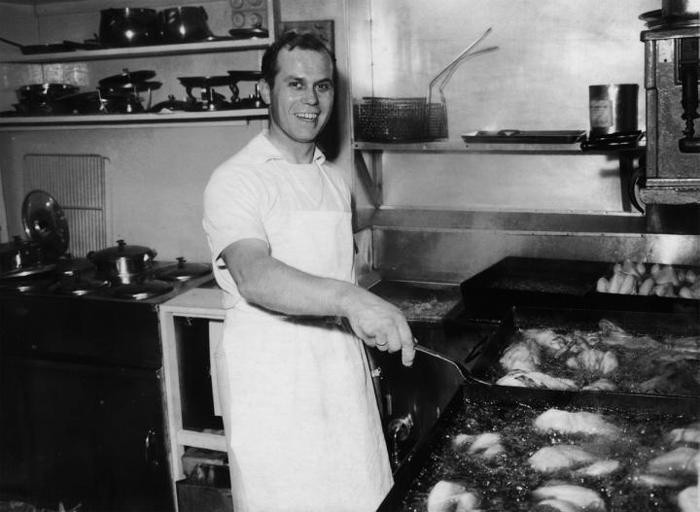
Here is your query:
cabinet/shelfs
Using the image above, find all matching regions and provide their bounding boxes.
[0,0,700,512]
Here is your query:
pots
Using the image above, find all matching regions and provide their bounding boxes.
[0,36,75,54]
[87,239,157,272]
[1,234,39,275]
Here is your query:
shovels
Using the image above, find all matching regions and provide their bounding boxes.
[414,343,495,386]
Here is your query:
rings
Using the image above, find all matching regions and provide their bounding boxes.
[375,339,390,348]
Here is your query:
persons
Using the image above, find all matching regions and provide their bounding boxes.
[200,30,421,511]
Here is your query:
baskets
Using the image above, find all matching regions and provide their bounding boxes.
[423,101,449,141]
[352,95,428,143]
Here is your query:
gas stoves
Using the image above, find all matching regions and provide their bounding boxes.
[32,255,214,299]
